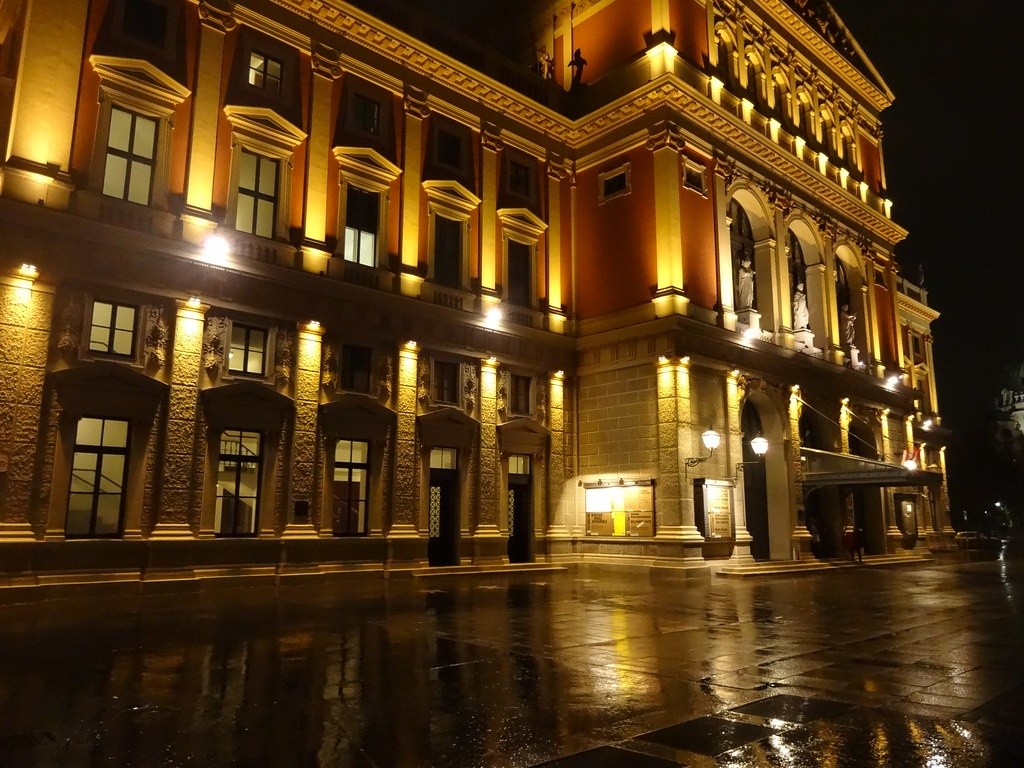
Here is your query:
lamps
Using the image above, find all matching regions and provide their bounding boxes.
[737,430,770,471]
[685,426,721,467]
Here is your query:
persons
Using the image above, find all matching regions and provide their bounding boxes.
[793,282,809,330]
[739,257,754,308]
[849,525,864,562]
[840,305,857,346]
